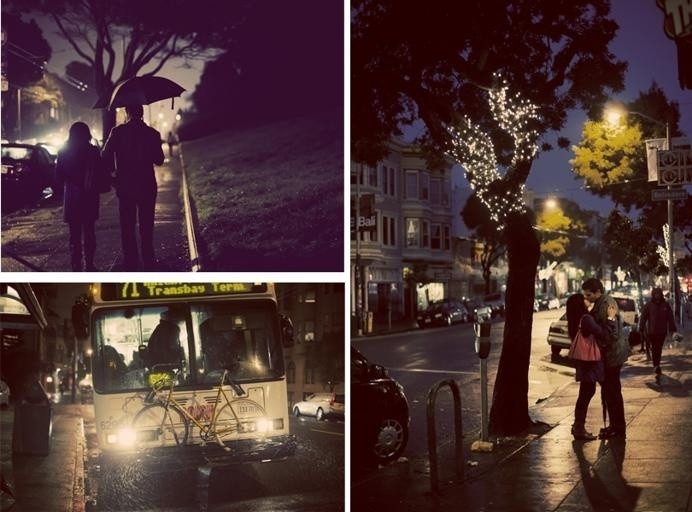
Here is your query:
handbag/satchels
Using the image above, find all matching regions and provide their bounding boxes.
[568,313,602,362]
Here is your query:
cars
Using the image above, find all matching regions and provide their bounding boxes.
[291,392,344,422]
[416,291,506,330]
[548,290,648,360]
[1,141,58,205]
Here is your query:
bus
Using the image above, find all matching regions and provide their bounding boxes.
[69,282,291,475]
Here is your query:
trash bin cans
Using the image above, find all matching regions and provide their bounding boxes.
[14,380,51,457]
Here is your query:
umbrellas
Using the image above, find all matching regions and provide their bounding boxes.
[89,74,185,113]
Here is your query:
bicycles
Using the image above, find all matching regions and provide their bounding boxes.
[129,359,273,457]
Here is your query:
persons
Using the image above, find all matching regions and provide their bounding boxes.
[50,119,114,271]
[97,102,166,274]
[639,287,680,376]
[580,276,634,441]
[166,130,177,158]
[144,303,187,380]
[564,293,620,445]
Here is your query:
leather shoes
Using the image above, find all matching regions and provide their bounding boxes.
[598,427,626,439]
[570,424,596,442]
